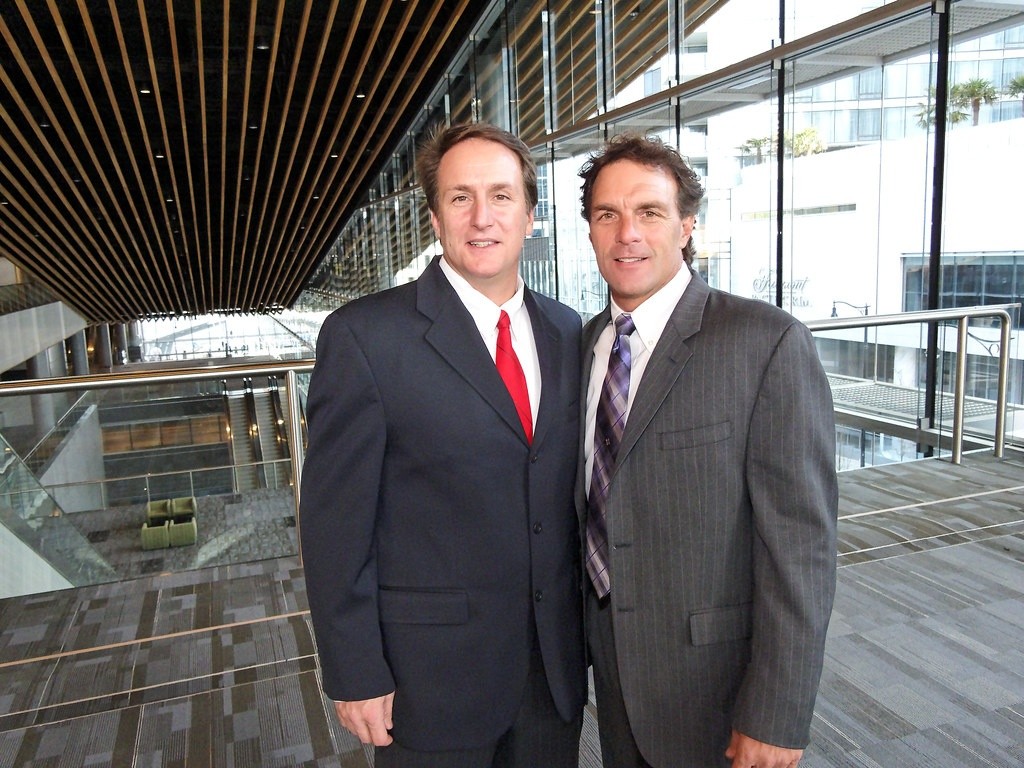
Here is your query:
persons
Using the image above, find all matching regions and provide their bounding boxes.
[300,122,593,768]
[576,131,839,768]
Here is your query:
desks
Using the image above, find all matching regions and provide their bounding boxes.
[151,515,190,527]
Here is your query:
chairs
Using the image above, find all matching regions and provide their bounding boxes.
[140,521,169,550]
[169,516,198,548]
[172,497,198,518]
[147,499,171,525]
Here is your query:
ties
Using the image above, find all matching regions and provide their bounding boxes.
[495,310,533,448]
[585,313,636,600]
